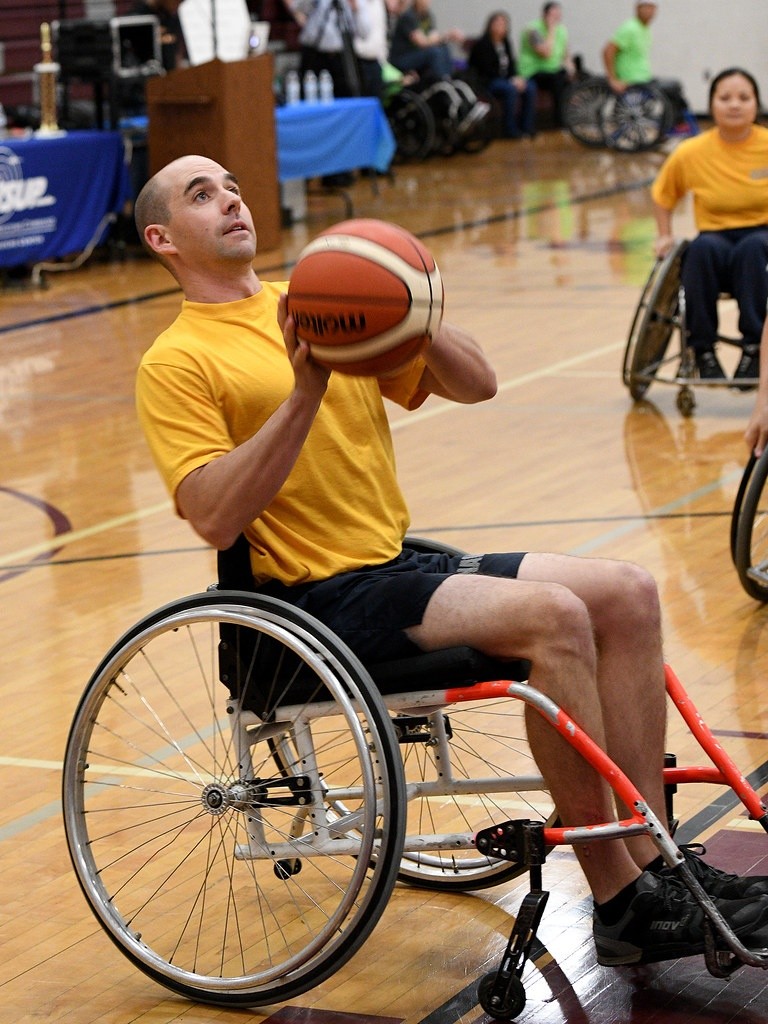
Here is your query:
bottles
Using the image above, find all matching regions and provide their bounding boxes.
[284,71,300,104]
[304,70,318,102]
[319,70,335,106]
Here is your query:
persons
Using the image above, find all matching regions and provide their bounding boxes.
[464,9,537,140]
[602,0,690,132]
[743,314,768,456]
[289,0,467,189]
[517,2,573,131]
[651,67,768,392]
[131,154,768,965]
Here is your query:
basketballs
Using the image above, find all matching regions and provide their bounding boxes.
[284,216,446,378]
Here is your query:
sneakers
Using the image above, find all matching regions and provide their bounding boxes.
[593,871,768,967]
[655,843,768,900]
[730,348,759,392]
[696,349,725,377]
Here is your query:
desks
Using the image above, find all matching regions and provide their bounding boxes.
[118,98,380,224]
[0,128,121,284]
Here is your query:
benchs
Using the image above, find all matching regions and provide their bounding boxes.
[465,38,553,132]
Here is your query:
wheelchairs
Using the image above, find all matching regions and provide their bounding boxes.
[62,533,768,1020]
[380,78,494,158]
[569,75,697,154]
[622,239,768,415]
[727,433,768,611]
